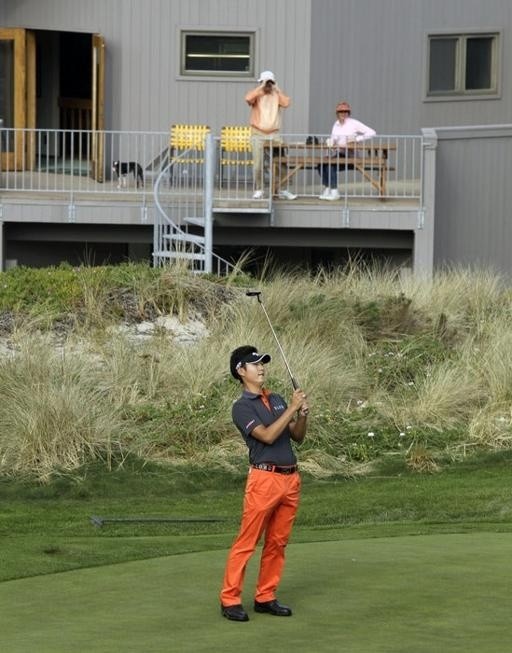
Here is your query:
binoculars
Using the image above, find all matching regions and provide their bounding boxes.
[306,136,318,145]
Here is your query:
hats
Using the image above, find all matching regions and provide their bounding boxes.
[335,102,351,116]
[230,345,272,379]
[257,70,276,82]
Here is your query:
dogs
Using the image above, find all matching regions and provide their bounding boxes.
[112,160,144,189]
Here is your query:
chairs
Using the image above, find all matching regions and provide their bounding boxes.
[168,124,212,189]
[218,126,252,190]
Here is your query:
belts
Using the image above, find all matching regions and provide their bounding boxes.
[252,462,299,474]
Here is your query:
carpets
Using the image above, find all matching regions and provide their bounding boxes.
[41,167,91,177]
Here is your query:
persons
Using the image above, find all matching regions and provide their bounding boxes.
[246,71,376,201]
[218,345,308,622]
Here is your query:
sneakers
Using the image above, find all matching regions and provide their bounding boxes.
[252,190,266,199]
[273,190,299,200]
[318,187,340,201]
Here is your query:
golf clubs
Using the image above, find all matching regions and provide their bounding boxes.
[246,292,309,415]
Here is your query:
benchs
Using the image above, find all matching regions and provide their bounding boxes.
[264,141,399,200]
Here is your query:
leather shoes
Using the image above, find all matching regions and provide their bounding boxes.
[221,603,249,621]
[253,600,292,616]
